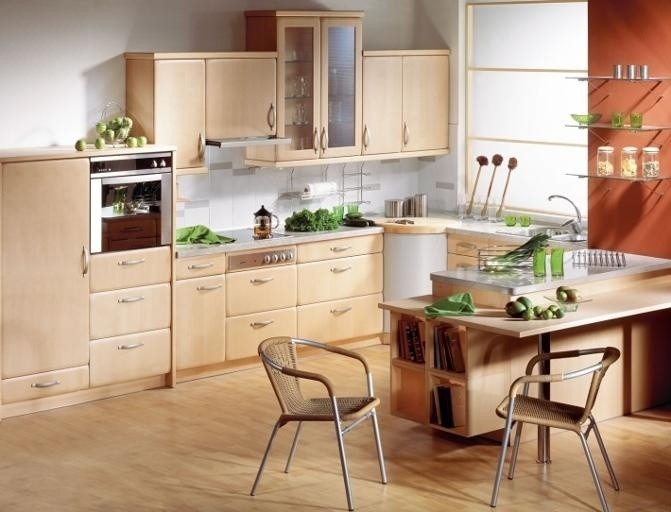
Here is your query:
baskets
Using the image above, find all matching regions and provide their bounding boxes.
[94,101,132,148]
[478,244,536,274]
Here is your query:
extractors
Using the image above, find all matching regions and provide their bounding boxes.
[207,136,294,147]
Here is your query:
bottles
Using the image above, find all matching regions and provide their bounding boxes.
[384,192,429,219]
[642,148,661,178]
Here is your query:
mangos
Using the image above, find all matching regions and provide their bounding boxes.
[516,297,532,312]
[564,289,578,302]
[556,286,571,300]
[504,301,527,317]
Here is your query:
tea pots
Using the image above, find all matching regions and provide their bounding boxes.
[254,205,280,235]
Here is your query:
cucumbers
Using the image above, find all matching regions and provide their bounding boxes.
[345,212,361,218]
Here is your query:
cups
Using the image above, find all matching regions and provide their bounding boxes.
[520,216,530,226]
[611,110,627,128]
[534,246,546,279]
[334,206,345,223]
[505,215,517,227]
[348,204,359,213]
[551,248,566,277]
[627,63,638,80]
[613,64,624,79]
[640,63,650,81]
[630,111,645,128]
[290,45,343,127]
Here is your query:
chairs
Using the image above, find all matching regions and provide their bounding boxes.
[490,346,621,512]
[247,336,390,512]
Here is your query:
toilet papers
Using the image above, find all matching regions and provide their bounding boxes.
[301,182,338,198]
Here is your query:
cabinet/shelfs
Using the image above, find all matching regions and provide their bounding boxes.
[124,52,280,177]
[390,310,513,441]
[1,156,91,418]
[91,245,173,401]
[244,10,365,168]
[447,233,527,271]
[362,49,450,161]
[564,76,670,197]
[228,267,297,372]
[176,255,233,375]
[297,232,384,354]
[101,216,161,252]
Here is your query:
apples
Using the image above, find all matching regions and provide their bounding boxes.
[75,140,86,150]
[96,117,133,140]
[127,137,137,147]
[137,136,147,147]
[95,138,105,149]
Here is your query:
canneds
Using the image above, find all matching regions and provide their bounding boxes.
[642,146,659,178]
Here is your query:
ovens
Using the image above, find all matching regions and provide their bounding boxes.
[91,150,173,254]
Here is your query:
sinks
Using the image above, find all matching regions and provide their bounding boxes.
[496,226,587,241]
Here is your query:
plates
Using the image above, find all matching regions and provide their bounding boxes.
[573,112,604,126]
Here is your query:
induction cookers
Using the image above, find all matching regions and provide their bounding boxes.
[215,227,297,273]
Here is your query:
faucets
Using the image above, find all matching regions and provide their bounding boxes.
[548,195,582,234]
